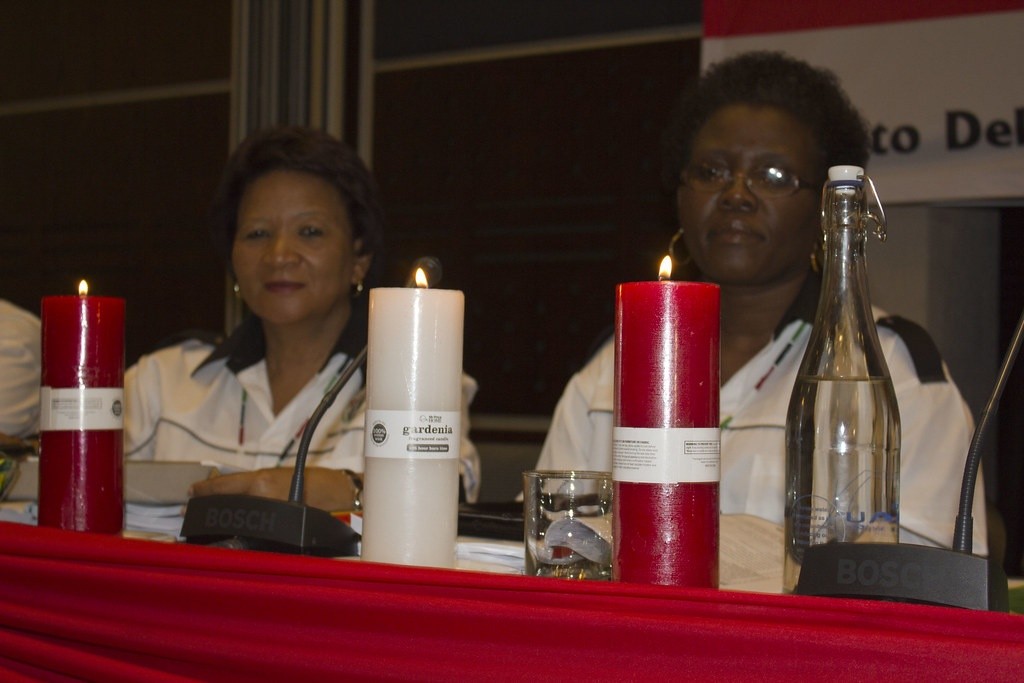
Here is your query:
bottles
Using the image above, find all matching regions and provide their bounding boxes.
[784,165,902,595]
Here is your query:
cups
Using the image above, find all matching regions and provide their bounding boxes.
[521,469,612,582]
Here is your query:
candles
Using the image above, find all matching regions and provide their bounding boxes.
[613,256,722,592]
[359,267,464,570]
[37,281,126,538]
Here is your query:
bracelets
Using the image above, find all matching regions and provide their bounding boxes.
[343,469,361,510]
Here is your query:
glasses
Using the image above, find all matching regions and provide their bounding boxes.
[671,159,822,198]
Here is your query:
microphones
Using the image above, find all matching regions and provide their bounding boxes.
[180,256,443,554]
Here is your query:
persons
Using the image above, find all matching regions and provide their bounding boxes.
[0,297,41,453]
[123,130,479,518]
[522,52,990,593]
